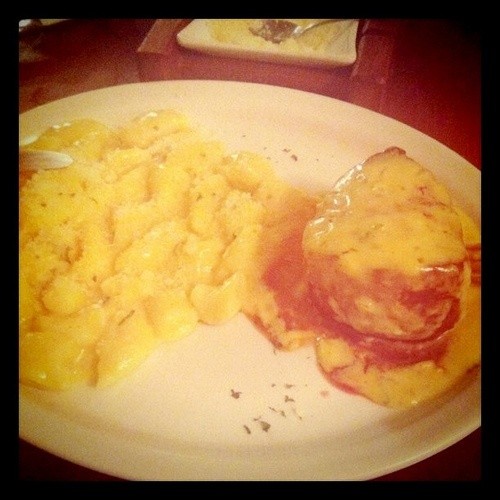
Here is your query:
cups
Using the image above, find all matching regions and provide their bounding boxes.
[19,19,43,62]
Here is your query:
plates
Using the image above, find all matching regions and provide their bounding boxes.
[18,79,482,481]
[177,19,359,66]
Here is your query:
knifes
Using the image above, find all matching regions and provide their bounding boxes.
[18,147,73,169]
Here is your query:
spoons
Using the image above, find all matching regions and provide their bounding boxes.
[248,19,350,44]
